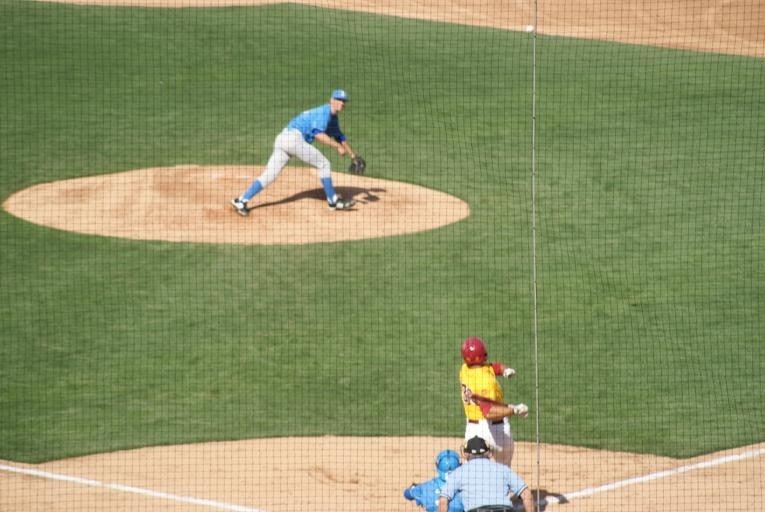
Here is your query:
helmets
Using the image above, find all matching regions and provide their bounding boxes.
[461,338,488,365]
[435,450,462,481]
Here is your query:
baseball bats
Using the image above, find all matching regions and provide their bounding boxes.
[466,393,517,410]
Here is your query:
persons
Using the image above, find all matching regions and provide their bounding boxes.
[404,449,465,512]
[438,435,535,512]
[459,338,528,469]
[230,88,365,217]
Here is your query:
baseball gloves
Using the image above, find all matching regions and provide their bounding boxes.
[347,155,367,176]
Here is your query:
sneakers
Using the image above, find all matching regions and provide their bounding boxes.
[328,198,355,210]
[230,198,248,216]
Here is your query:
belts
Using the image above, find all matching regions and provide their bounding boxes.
[466,420,505,425]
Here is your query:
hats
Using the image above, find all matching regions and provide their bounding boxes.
[332,89,348,101]
[465,435,489,455]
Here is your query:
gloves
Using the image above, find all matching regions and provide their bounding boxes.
[514,402,528,419]
[504,367,516,382]
